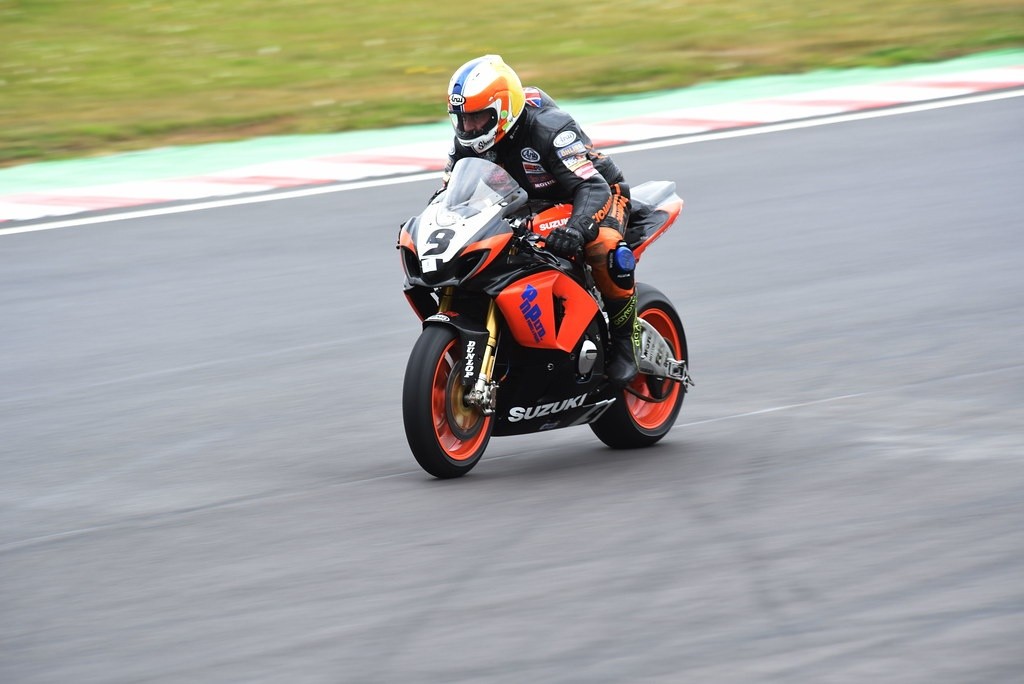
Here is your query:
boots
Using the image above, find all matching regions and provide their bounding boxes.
[601,286,641,387]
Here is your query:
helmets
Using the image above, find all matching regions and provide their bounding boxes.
[447,54,526,154]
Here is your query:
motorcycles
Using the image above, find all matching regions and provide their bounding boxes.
[396,157,693,481]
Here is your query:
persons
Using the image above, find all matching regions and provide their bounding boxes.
[426,55,642,382]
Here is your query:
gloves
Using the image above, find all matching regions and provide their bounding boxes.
[545,215,599,262]
